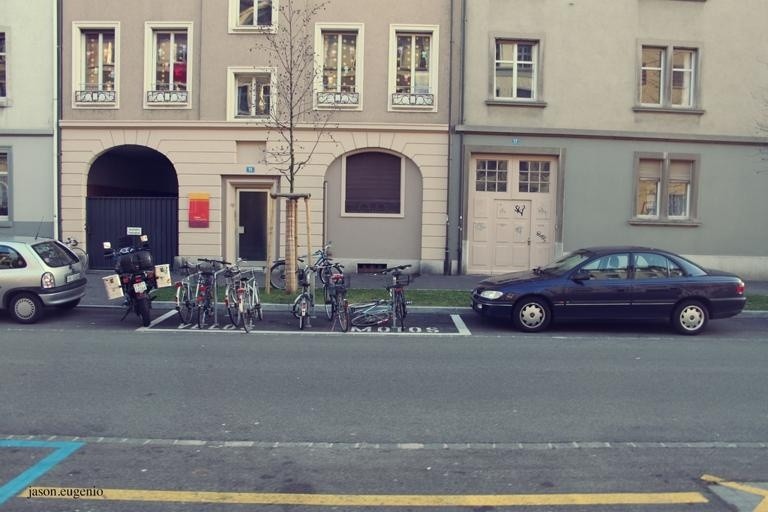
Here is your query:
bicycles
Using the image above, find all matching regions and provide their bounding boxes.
[57,237,88,269]
[175,241,413,334]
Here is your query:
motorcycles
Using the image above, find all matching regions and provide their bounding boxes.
[101,235,172,329]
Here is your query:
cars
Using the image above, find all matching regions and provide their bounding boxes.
[466,245,746,335]
[0,215,87,323]
